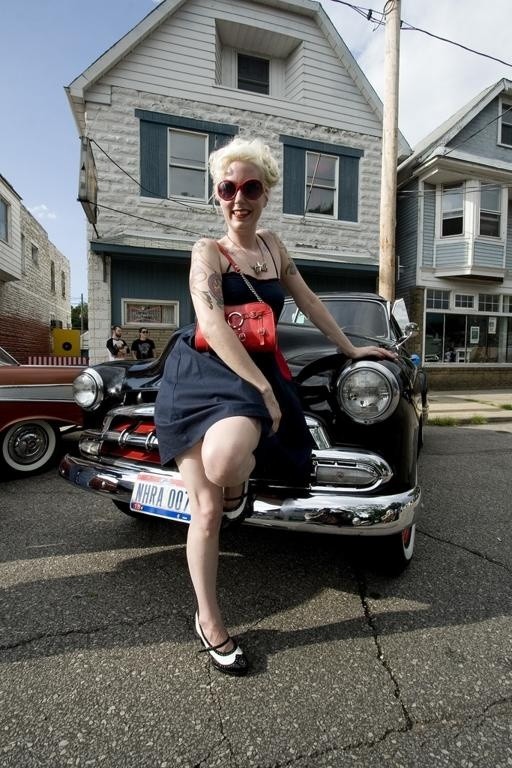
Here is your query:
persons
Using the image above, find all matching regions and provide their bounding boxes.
[129,327,158,360]
[105,325,131,361]
[152,132,399,677]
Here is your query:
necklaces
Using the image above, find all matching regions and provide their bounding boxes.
[226,232,269,276]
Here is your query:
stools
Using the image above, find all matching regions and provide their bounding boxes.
[454,347,471,363]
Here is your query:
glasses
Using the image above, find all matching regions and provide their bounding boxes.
[216,177,270,202]
[141,331,148,334]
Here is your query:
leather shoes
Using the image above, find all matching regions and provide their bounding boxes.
[193,609,249,677]
[218,474,250,523]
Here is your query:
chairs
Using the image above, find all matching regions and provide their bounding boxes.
[473,346,498,362]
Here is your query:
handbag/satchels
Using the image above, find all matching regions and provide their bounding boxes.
[193,240,279,354]
